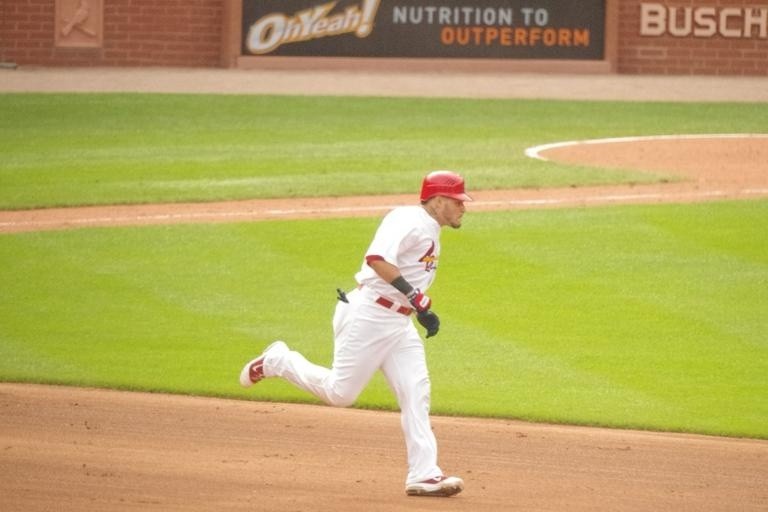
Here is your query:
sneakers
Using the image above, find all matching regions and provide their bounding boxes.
[405,476,465,497]
[239,341,288,388]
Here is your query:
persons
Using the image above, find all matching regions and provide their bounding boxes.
[237,169,472,497]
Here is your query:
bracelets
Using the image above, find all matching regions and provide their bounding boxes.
[389,275,413,295]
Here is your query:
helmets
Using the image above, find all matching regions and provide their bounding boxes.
[420,170,473,202]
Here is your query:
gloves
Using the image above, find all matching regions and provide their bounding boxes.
[336,288,349,303]
[407,288,440,339]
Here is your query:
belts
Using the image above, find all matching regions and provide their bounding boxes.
[357,284,412,316]
